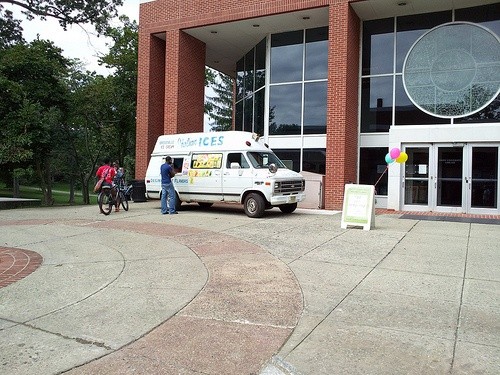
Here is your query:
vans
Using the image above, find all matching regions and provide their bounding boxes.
[144,131,306,218]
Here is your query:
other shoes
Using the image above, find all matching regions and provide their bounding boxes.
[114,208,119,212]
[105,207,110,210]
[163,212,169,214]
[171,211,179,214]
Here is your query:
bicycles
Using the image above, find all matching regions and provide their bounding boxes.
[98,176,129,215]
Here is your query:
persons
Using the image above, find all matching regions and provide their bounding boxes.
[160,156,178,215]
[94,158,125,211]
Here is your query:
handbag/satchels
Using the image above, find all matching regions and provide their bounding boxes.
[94,178,104,193]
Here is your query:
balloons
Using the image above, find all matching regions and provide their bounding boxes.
[390,148,400,159]
[385,153,395,164]
[396,152,408,164]
